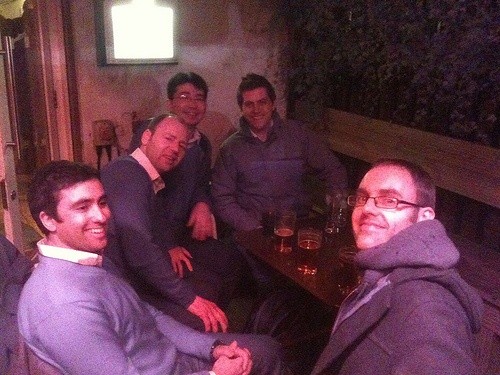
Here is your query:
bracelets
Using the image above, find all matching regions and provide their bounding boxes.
[209,341,227,365]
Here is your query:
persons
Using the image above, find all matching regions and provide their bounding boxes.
[92,113,232,334]
[211,73,348,296]
[309,157,481,375]
[16,159,284,375]
[130,72,257,311]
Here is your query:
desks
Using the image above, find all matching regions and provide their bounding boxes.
[236,214,498,372]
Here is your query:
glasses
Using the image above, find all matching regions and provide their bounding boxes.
[166,93,209,105]
[347,194,423,211]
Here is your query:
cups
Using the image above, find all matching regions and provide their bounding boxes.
[337,246,361,293]
[263,201,277,237]
[326,195,352,234]
[274,209,296,252]
[297,227,323,274]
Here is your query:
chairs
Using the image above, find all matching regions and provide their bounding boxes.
[19,339,74,374]
